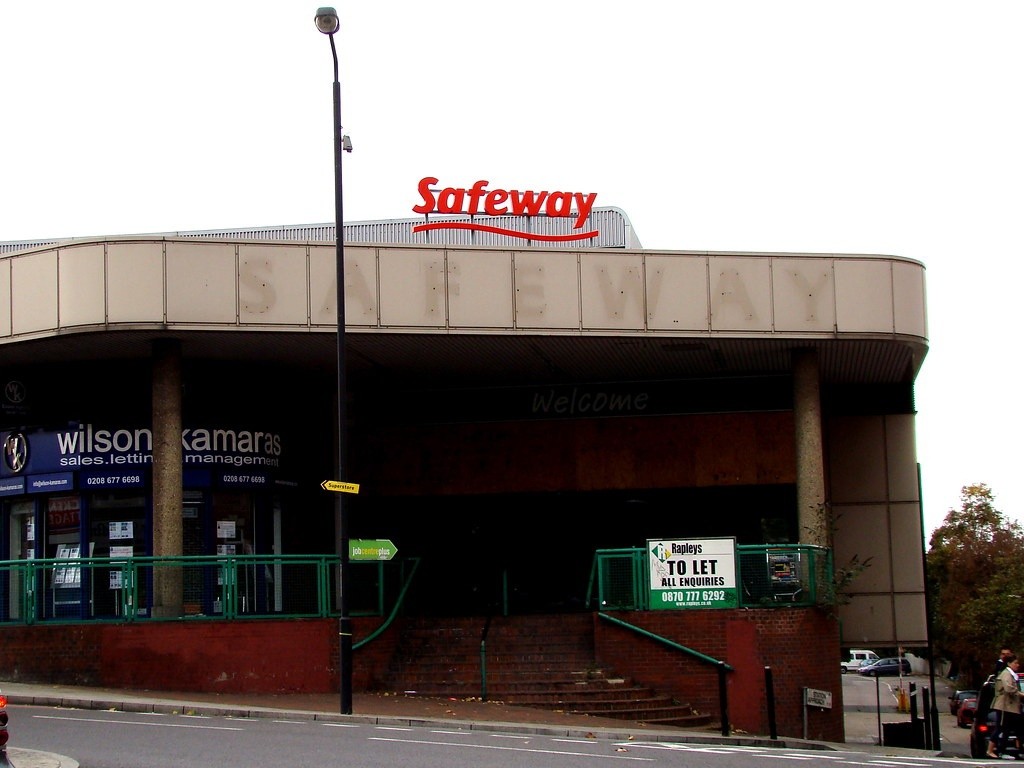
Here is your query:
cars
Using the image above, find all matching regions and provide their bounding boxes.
[856,657,912,677]
[955,698,978,728]
[860,658,878,669]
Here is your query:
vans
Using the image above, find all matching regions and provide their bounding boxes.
[841,649,880,674]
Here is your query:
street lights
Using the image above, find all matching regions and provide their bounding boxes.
[314,7,360,719]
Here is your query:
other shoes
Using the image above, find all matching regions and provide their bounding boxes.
[985,752,1001,759]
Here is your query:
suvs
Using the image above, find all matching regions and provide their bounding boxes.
[968,672,1024,759]
[948,689,980,716]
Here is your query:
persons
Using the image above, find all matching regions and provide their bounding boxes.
[986,646,1024,760]
